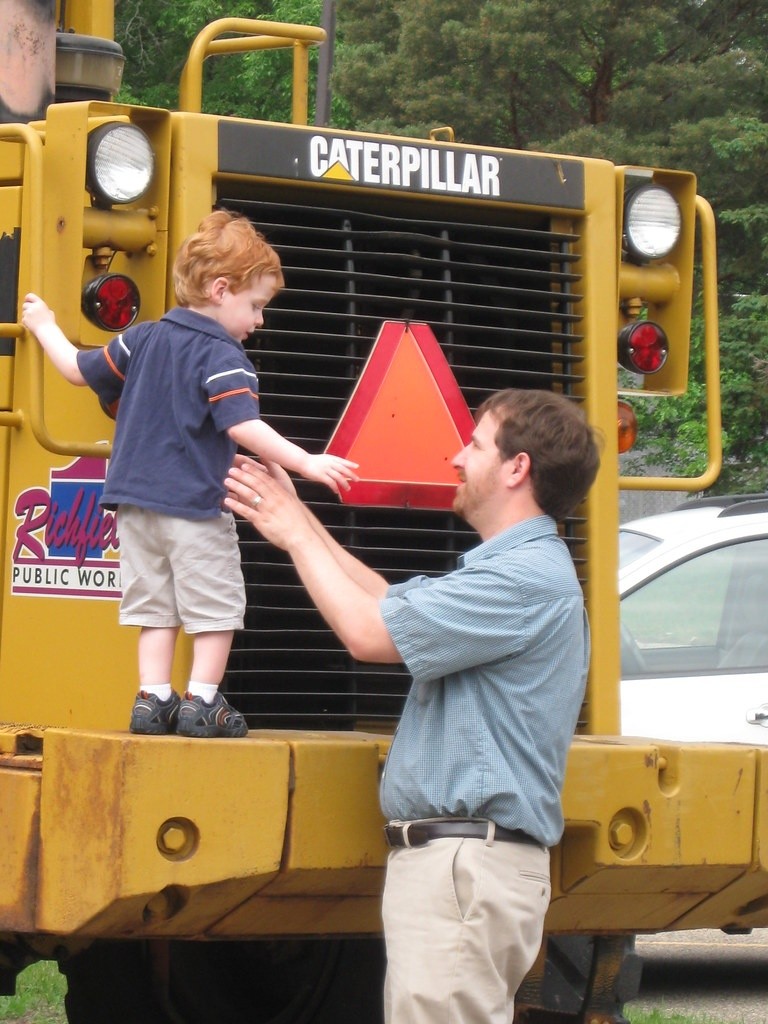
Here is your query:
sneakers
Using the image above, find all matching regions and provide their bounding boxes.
[131,688,182,734]
[177,690,249,737]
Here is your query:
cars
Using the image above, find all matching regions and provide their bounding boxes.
[621,493,768,745]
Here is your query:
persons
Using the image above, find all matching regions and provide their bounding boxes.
[21,211,360,738]
[224,388,600,1024]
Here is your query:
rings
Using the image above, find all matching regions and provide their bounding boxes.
[253,496,261,506]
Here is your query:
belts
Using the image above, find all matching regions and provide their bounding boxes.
[383,815,540,849]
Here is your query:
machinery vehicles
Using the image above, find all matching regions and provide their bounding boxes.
[0,0,768,1024]
